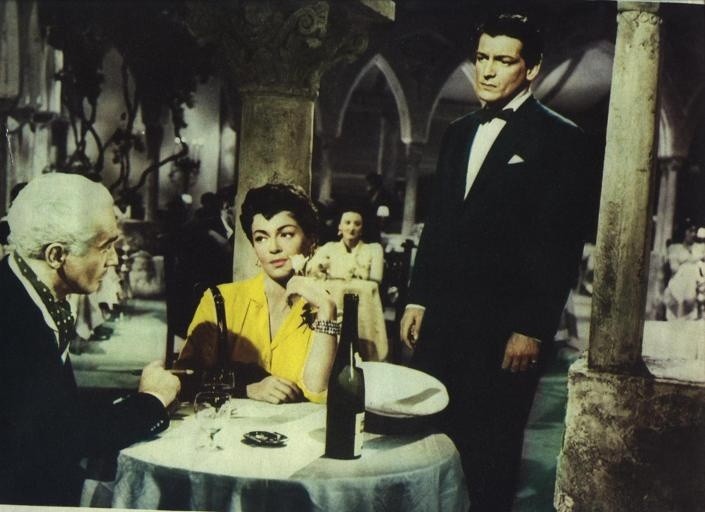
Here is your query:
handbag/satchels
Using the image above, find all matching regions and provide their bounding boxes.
[173,356,269,407]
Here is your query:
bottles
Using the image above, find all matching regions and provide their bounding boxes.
[324,292,365,460]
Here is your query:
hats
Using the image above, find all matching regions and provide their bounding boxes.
[355,361,450,434]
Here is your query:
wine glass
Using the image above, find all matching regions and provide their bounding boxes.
[192,367,236,452]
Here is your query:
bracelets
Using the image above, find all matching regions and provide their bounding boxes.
[313,320,341,335]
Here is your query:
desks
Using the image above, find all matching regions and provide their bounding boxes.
[105,398,461,509]
[314,273,388,359]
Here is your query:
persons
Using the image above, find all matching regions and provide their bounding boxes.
[175,181,338,406]
[0,11,704,507]
[186,186,238,281]
[401,15,589,506]
[0,182,28,245]
[304,207,383,283]
[652,236,705,321]
[0,173,180,507]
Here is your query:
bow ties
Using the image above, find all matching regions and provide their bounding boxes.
[476,108,513,124]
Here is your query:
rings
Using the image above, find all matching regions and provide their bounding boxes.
[529,360,536,368]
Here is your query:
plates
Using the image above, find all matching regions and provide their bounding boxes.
[243,430,287,446]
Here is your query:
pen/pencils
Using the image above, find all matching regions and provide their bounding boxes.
[131,369,194,376]
